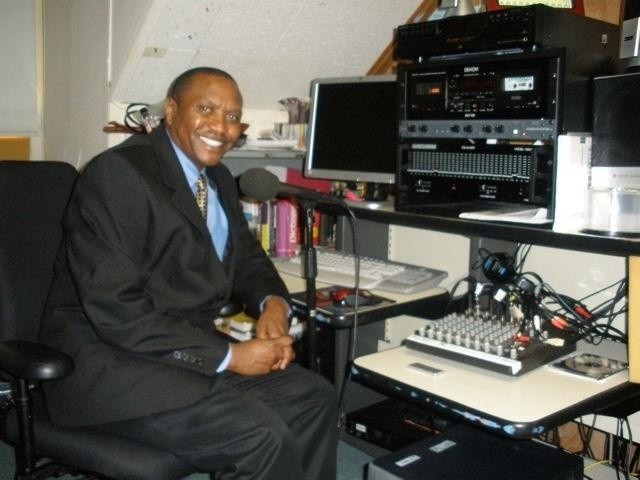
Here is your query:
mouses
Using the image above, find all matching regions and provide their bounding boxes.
[332,286,374,306]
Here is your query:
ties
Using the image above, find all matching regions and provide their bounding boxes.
[196,174,207,225]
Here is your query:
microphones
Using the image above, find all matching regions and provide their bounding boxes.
[236,166,337,210]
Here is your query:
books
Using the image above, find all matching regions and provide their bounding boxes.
[220,309,300,335]
[240,97,311,152]
[212,312,308,343]
[239,164,337,260]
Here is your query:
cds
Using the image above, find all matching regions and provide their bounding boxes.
[563,353,625,376]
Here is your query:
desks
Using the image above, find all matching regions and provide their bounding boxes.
[259,180,640,478]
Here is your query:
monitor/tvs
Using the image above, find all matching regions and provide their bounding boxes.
[302,74,396,198]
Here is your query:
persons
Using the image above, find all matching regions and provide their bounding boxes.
[38,64,345,478]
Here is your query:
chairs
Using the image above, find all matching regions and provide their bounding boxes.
[0,158,198,478]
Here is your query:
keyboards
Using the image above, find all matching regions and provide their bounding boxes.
[273,244,447,293]
